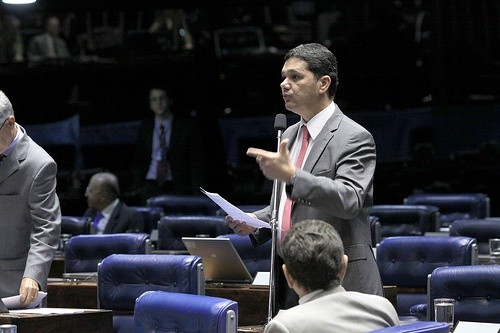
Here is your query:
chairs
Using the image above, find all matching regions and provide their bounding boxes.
[60,193,500,333]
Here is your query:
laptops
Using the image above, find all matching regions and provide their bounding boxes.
[182,237,253,284]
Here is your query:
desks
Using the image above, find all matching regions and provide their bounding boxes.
[48,281,276,325]
[0,308,113,333]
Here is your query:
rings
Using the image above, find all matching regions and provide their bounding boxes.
[31,296,35,299]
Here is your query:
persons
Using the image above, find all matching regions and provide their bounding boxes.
[0,91,61,310]
[73,173,146,236]
[226,43,385,310]
[0,0,500,225]
[264,219,402,333]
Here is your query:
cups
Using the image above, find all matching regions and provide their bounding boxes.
[434,298,455,333]
[489,239,500,257]
[59,235,72,254]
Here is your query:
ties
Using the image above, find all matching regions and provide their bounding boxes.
[92,212,103,234]
[156,124,168,187]
[281,125,310,243]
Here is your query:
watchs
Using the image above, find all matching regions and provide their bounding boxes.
[287,172,297,186]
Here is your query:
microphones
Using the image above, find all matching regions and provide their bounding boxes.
[275,114,288,152]
